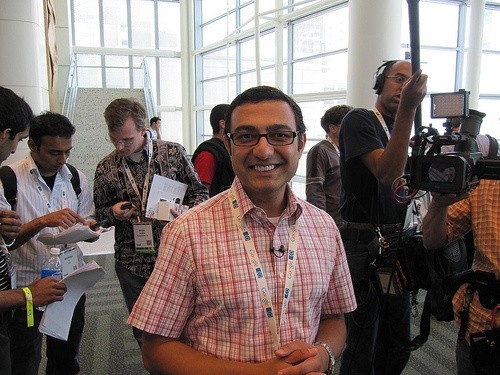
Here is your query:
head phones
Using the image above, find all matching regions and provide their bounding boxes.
[373,60,397,95]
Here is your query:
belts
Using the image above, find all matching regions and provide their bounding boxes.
[343,220,404,232]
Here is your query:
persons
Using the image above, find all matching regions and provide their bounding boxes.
[0,111,100,375]
[0,86,67,375]
[93,98,209,353]
[409,118,500,321]
[422,181,500,375]
[125,85,357,375]
[306,104,354,230]
[191,104,231,198]
[340,59,428,375]
[146,117,162,140]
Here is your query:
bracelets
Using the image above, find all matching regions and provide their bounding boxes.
[22,286,35,327]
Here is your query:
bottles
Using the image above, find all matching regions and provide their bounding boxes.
[35,248,62,312]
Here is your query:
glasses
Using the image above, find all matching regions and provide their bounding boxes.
[226,130,301,148]
[104,130,141,145]
[386,74,409,86]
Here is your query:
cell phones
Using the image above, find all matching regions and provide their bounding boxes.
[90,221,103,231]
[121,202,133,211]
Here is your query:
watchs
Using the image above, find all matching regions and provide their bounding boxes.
[312,342,336,375]
